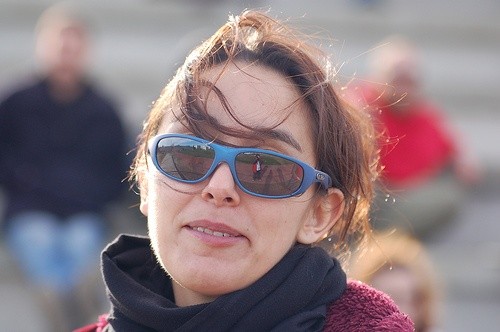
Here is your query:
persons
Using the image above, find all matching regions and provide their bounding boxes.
[73,6,417,332]
[330,41,478,249]
[252,153,263,181]
[1,0,133,305]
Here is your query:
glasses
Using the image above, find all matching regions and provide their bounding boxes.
[146,134,331,199]
